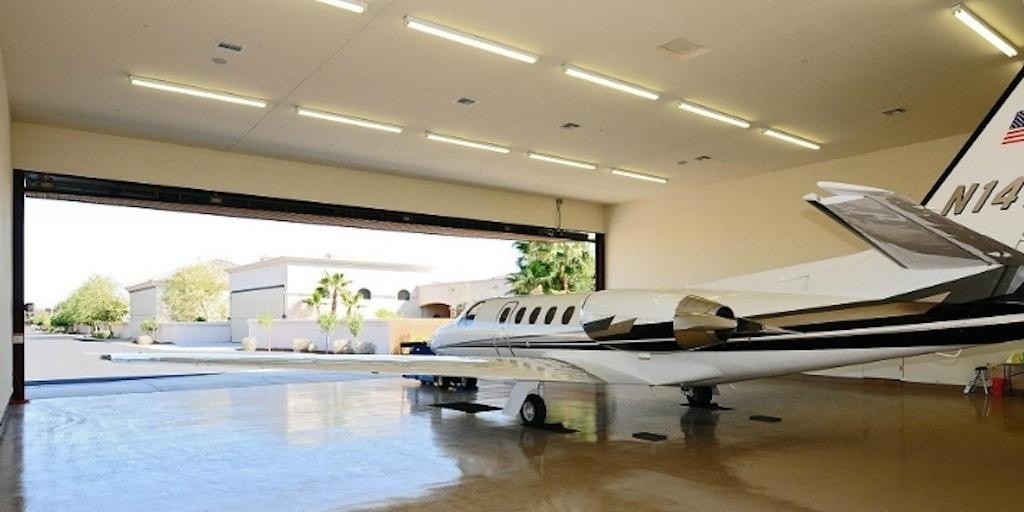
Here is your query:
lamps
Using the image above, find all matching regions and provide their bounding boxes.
[675,97,751,130]
[422,127,511,157]
[401,13,541,66]
[760,123,822,154]
[948,2,1019,58]
[316,0,370,17]
[291,105,404,137]
[125,72,270,110]
[558,58,661,105]
[525,150,599,170]
[607,166,670,184]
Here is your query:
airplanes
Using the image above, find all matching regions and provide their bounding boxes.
[98,64,1023,429]
